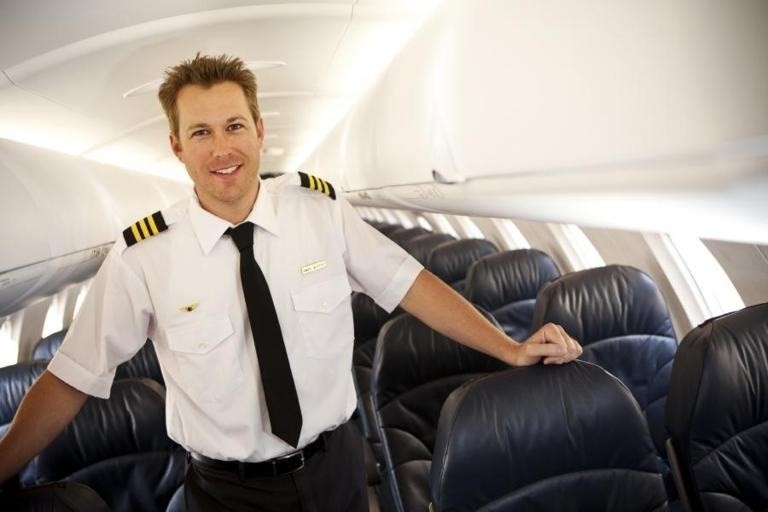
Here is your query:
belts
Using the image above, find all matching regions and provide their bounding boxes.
[190,430,332,474]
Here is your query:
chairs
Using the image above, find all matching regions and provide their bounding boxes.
[0,329,190,512]
[426,234,499,300]
[669,299,766,512]
[531,263,683,468]
[362,227,431,252]
[409,229,459,272]
[372,296,508,512]
[421,355,682,511]
[354,291,393,438]
[462,246,563,344]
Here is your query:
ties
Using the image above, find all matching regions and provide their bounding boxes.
[224,223,303,448]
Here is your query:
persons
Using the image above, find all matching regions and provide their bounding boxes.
[0,56,585,511]
